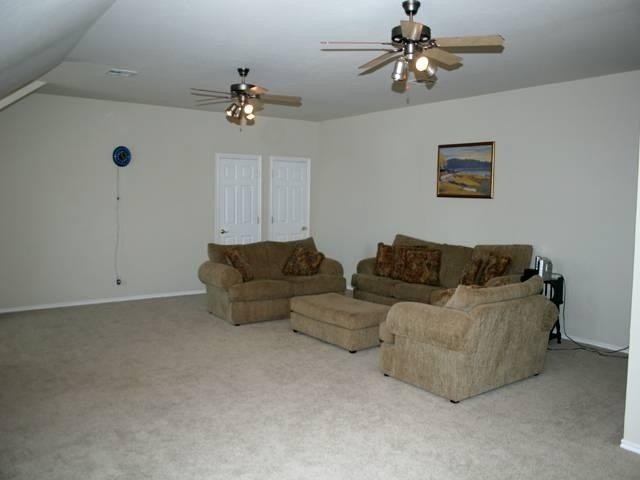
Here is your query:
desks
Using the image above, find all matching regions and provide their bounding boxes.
[288,291,390,353]
[540,273,564,343]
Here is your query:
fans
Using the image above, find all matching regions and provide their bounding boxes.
[190,68,303,108]
[322,1,506,69]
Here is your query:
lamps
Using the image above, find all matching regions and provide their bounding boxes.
[392,45,437,81]
[226,95,255,127]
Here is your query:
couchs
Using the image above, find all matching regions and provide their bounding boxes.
[377,275,557,405]
[200,236,348,326]
[352,232,532,304]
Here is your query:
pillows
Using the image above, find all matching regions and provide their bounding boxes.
[221,243,255,281]
[283,241,322,277]
[462,250,515,287]
[374,243,441,286]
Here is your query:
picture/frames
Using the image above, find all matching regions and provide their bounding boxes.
[435,140,496,198]
